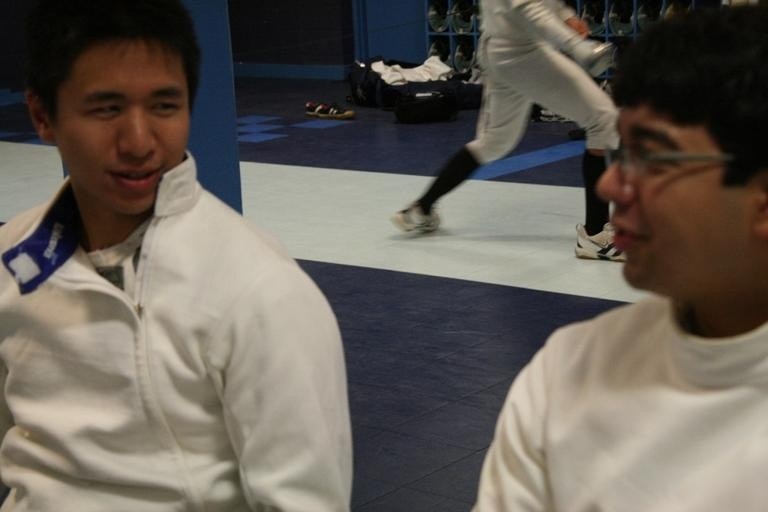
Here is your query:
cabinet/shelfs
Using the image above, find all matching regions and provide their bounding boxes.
[422,1,668,82]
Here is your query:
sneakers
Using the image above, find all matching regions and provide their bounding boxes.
[396,206,439,232]
[575,224,628,261]
[306,102,353,118]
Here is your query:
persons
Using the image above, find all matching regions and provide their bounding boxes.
[392,1,626,260]
[474,1,768,510]
[2,0,352,512]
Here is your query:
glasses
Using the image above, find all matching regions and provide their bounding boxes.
[602,144,730,172]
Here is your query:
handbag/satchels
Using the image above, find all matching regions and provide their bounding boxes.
[350,57,458,123]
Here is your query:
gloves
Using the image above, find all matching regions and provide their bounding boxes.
[572,40,600,62]
